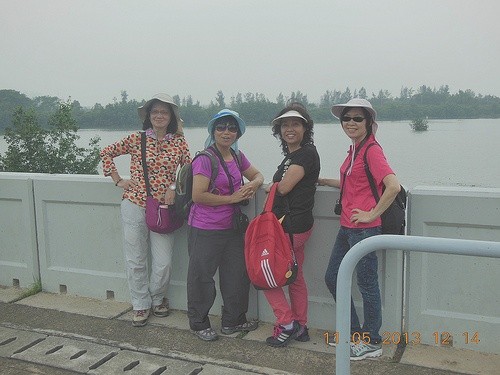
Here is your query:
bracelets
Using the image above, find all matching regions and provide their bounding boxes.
[115,178,123,186]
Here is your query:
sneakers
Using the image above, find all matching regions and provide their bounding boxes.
[131,310,151,327]
[221,320,260,335]
[194,327,218,341]
[266,320,300,347]
[326,335,383,361]
[151,305,169,317]
[291,326,310,342]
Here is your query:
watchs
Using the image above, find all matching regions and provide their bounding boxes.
[168,185,177,192]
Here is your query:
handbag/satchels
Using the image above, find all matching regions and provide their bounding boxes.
[145,192,185,234]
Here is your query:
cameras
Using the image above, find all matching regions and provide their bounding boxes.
[238,198,249,206]
[334,203,342,215]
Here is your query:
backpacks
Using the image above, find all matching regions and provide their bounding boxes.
[363,142,406,235]
[244,182,298,290]
[164,147,242,219]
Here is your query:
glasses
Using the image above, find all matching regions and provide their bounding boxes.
[340,116,366,122]
[214,126,239,133]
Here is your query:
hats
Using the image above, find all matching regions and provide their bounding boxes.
[137,93,184,135]
[272,109,308,126]
[330,98,378,135]
[204,109,246,155]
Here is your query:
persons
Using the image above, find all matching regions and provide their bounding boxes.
[187,109,264,342]
[265,105,321,347]
[318,98,401,359]
[99,92,192,327]
